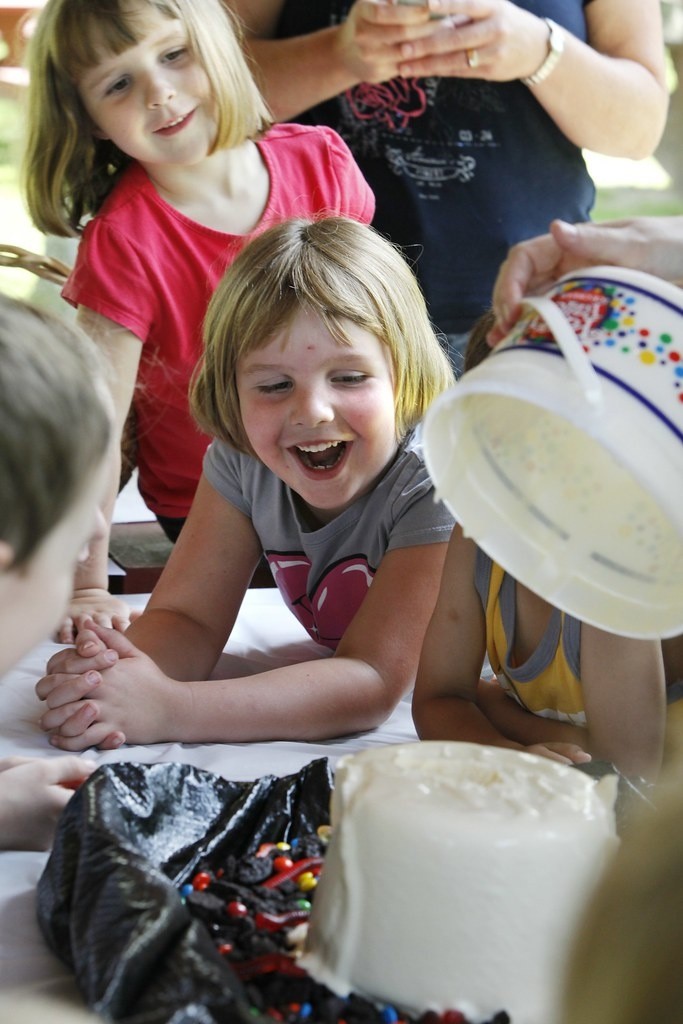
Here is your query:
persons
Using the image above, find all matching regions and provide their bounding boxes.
[0,292,118,855]
[406,516,683,789]
[217,0,669,379]
[37,209,460,750]
[19,0,378,544]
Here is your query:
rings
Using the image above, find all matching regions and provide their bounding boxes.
[466,48,479,68]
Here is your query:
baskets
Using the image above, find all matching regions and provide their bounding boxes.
[0,244,140,495]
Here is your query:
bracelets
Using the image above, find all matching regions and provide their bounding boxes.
[523,18,565,87]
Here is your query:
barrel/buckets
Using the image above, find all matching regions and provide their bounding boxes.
[419,264,683,642]
